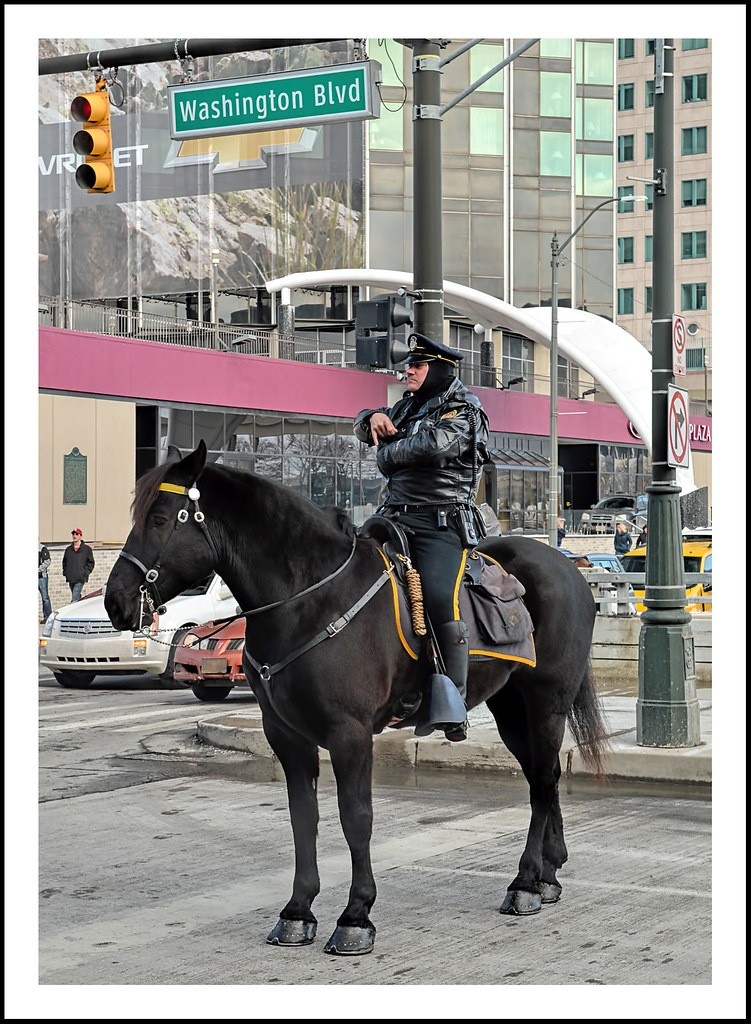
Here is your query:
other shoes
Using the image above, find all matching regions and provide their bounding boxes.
[40,619,47,624]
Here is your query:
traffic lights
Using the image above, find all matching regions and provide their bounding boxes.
[64,80,117,202]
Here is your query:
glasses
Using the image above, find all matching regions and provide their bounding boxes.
[73,533,80,535]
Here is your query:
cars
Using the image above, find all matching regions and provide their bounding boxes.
[172,613,253,704]
[557,548,573,557]
[566,553,636,605]
[623,537,713,613]
[37,574,242,690]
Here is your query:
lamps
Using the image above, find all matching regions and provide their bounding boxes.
[219,333,255,352]
[573,387,600,400]
[495,377,527,390]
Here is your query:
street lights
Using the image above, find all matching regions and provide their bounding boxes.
[542,191,650,550]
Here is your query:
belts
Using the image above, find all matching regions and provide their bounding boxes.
[384,504,438,513]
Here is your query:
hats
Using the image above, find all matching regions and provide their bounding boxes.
[71,528,82,534]
[405,333,463,368]
[617,524,627,533]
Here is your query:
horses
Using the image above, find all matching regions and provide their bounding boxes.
[105,439,612,958]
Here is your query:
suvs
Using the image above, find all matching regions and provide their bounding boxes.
[581,494,649,535]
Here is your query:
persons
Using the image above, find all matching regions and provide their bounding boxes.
[39,544,52,624]
[556,520,565,547]
[574,558,590,567]
[62,528,94,602]
[614,523,632,555]
[353,333,489,743]
[636,524,648,548]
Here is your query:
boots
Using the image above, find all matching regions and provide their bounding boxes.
[428,620,469,742]
